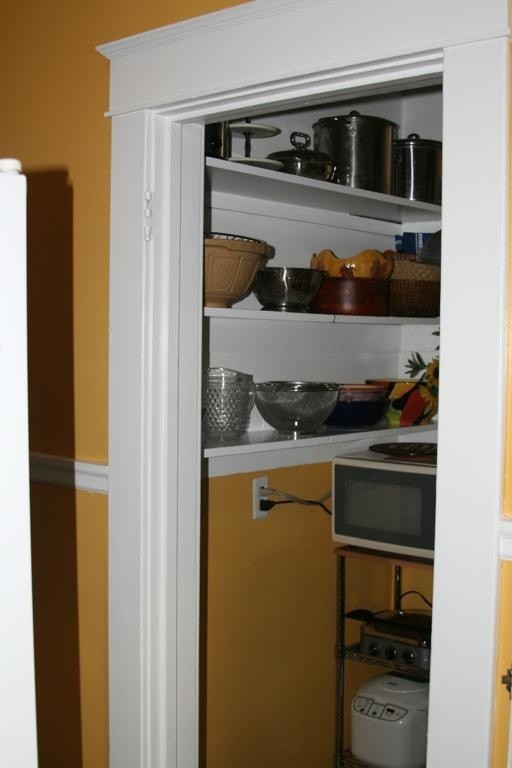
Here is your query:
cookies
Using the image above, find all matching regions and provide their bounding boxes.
[329,448,436,560]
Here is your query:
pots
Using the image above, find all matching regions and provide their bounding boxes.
[267,107,442,207]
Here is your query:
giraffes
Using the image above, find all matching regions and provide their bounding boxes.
[355,610,431,680]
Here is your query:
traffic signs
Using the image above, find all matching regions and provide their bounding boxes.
[250,475,272,517]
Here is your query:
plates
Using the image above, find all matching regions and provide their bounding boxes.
[331,534,433,766]
[201,155,442,461]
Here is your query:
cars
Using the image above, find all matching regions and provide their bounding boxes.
[204,121,230,160]
[202,367,256,444]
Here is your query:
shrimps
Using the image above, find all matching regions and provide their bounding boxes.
[348,672,429,768]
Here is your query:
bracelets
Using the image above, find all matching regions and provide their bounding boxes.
[252,373,434,432]
[251,264,438,319]
[204,238,275,309]
